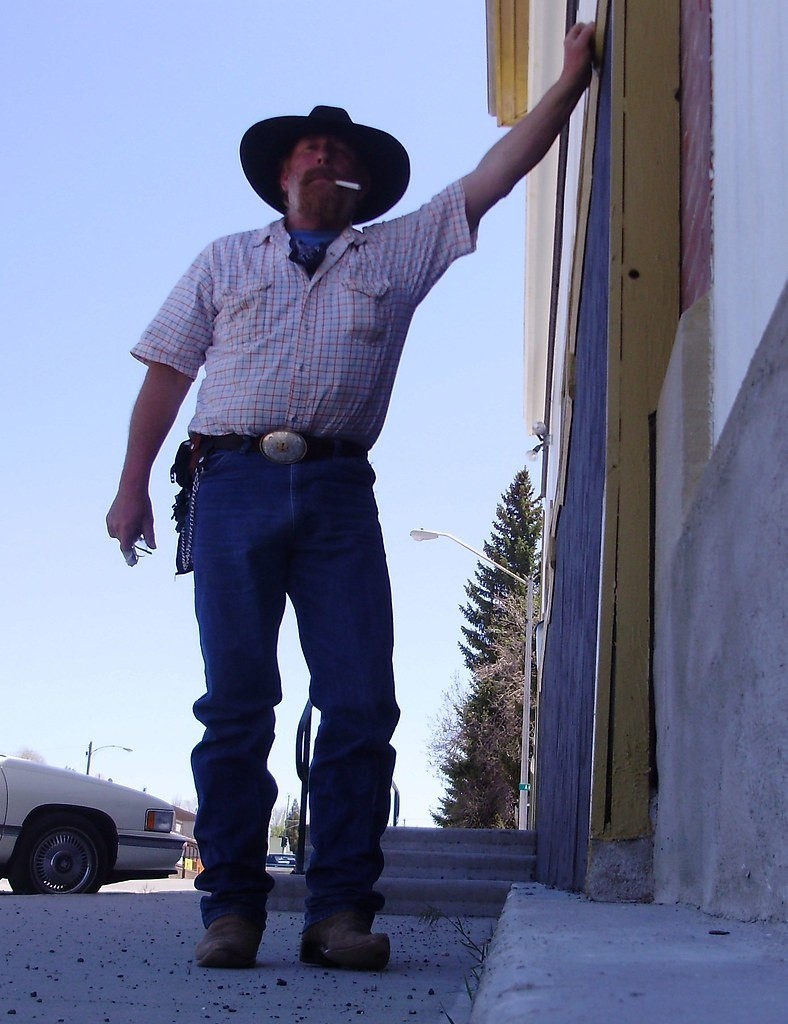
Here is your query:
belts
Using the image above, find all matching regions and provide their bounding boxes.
[214,434,351,457]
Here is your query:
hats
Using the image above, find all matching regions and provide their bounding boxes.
[238,106,410,225]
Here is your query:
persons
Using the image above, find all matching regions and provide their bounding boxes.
[105,20,595,971]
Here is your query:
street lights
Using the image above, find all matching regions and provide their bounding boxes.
[410,527,535,831]
[85,741,133,776]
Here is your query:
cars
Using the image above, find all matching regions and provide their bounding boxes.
[266,853,296,868]
[0,754,187,894]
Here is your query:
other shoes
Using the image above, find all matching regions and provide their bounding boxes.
[300,912,390,969]
[195,914,262,969]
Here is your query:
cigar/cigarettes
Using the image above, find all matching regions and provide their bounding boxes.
[332,180,362,191]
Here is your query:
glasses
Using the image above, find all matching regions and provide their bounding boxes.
[132,538,153,556]
